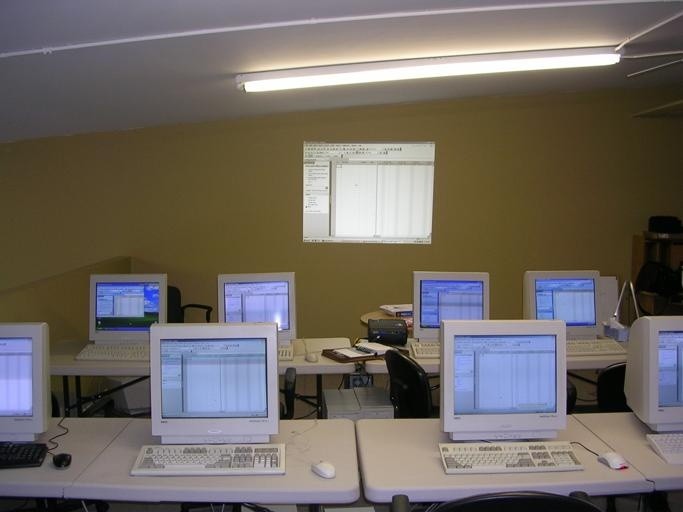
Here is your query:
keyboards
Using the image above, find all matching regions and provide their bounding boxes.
[437,441,584,475]
[1,441,48,468]
[566,338,626,355]
[645,433,683,464]
[280,348,294,361]
[410,342,440,359]
[74,345,149,362]
[129,445,287,475]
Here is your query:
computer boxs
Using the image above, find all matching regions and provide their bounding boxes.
[355,385,394,419]
[322,389,360,420]
[346,374,374,388]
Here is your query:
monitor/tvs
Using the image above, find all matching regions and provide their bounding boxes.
[218,272,297,343]
[624,315,683,431]
[150,322,279,444]
[1,322,52,440]
[440,318,566,440]
[88,272,168,343]
[412,270,490,341]
[522,270,601,340]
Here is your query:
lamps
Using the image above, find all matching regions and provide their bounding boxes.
[231,38,629,98]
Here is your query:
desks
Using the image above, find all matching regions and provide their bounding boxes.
[0,413,134,512]
[275,335,354,416]
[572,405,682,497]
[61,410,361,512]
[353,412,654,512]
[359,308,412,333]
[364,327,624,378]
[48,349,150,417]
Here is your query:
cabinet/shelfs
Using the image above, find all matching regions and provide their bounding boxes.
[628,231,682,321]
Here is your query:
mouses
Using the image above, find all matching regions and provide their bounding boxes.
[312,460,337,479]
[305,353,319,363]
[53,452,72,469]
[598,450,627,470]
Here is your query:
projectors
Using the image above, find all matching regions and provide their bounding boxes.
[367,318,408,346]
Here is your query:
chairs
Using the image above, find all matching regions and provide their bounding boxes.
[565,372,579,414]
[594,359,624,412]
[277,365,298,420]
[166,283,213,325]
[425,491,608,512]
[382,345,439,419]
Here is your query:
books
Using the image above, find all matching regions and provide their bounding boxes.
[322,339,403,362]
